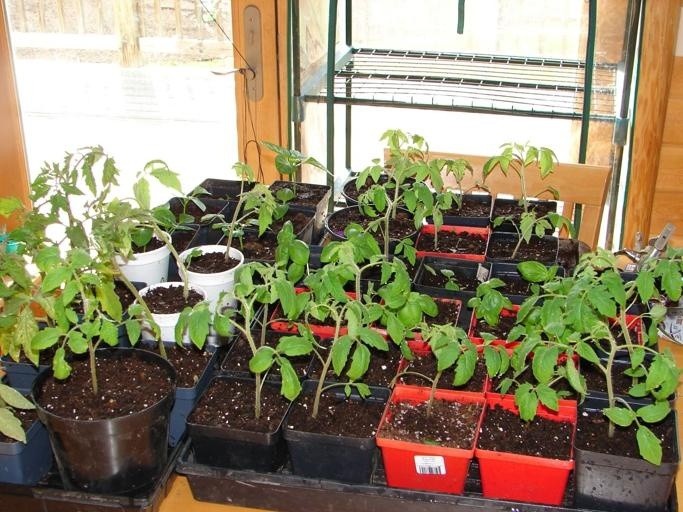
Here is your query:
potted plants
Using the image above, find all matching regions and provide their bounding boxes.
[30,140,146,346]
[103,128,683,512]
[0,193,67,487]
[1,130,185,495]
